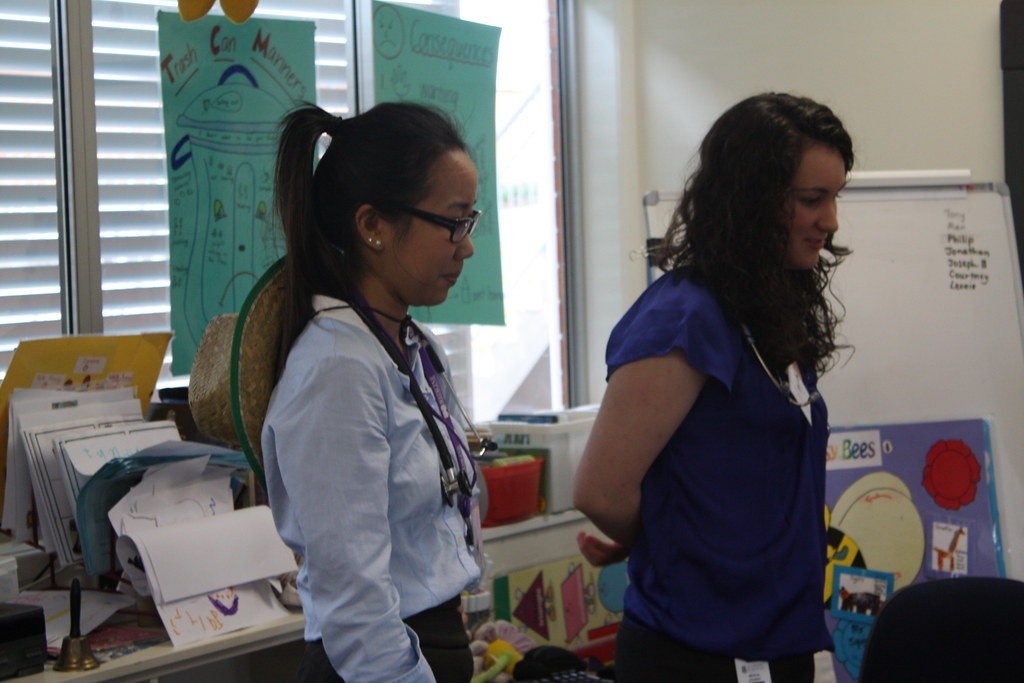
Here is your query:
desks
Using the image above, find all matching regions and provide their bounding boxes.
[477,508,606,574]
[0,608,305,683]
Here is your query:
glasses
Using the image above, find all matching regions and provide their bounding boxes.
[403,208,484,244]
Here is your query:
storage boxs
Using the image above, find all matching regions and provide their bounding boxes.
[480,412,598,528]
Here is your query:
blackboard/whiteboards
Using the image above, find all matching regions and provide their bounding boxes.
[644,180,1024,581]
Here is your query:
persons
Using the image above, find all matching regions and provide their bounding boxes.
[258,99,491,683]
[572,90,858,683]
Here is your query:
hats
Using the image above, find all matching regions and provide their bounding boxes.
[189,254,295,501]
